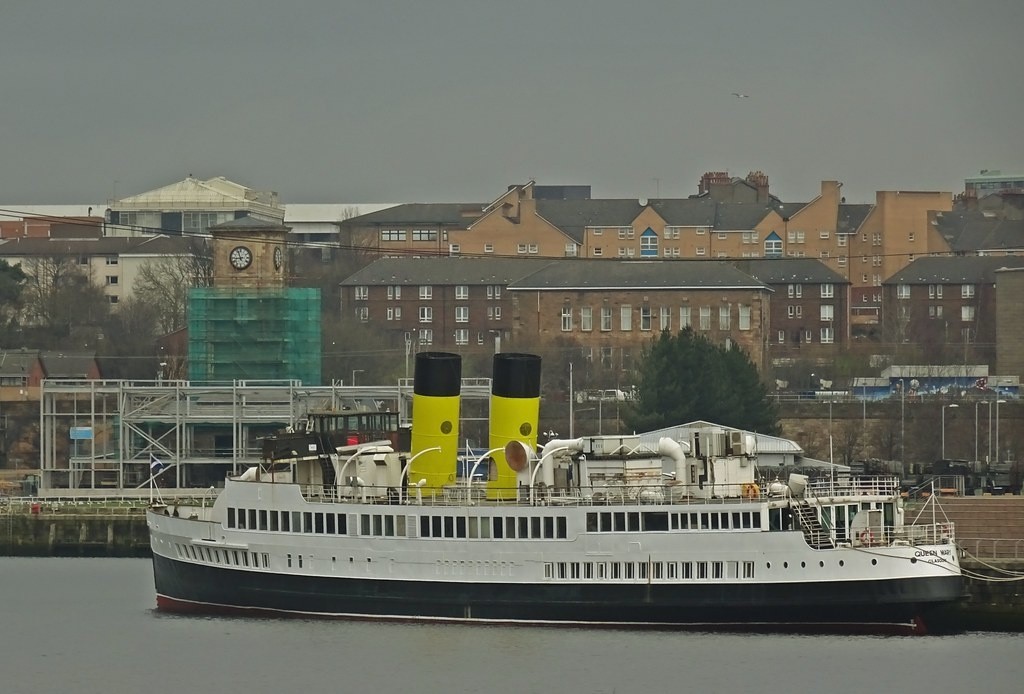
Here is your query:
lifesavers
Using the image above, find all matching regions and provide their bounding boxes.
[860,530,874,546]
[942,524,949,533]
[741,482,759,500]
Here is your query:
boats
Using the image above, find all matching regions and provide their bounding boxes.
[142,348,971,632]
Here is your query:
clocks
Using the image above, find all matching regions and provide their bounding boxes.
[273,247,281,271]
[230,246,253,270]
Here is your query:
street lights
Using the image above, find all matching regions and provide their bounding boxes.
[899,378,904,479]
[862,382,867,460]
[974,400,988,460]
[352,370,365,386]
[994,379,1013,462]
[941,403,959,461]
